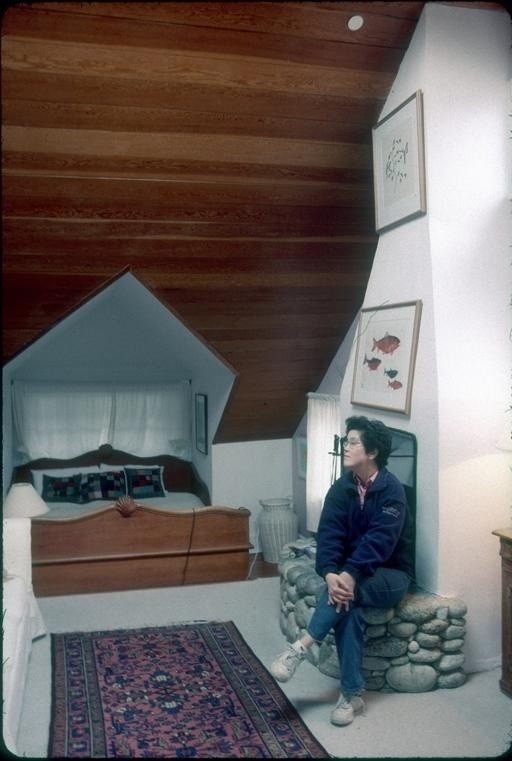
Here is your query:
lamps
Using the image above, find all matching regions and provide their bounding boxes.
[3,483,49,523]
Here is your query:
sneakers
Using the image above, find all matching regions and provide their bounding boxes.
[331,693,365,724]
[271,641,308,682]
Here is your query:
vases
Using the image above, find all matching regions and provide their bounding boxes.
[256,499,299,565]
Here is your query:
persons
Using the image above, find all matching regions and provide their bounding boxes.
[267,416,417,727]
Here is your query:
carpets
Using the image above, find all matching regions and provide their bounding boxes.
[49,620,331,760]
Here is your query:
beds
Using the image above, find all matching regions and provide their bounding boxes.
[3,445,253,598]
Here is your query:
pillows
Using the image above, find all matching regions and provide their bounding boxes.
[29,463,171,502]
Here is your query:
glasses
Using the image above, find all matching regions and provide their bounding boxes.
[343,441,364,449]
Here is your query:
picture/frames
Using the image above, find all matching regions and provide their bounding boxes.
[349,299,424,419]
[369,88,427,235]
[194,393,207,456]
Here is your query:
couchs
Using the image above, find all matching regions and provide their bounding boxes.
[3,519,43,758]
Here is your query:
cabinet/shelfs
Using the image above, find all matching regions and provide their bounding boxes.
[492,527,512,699]
